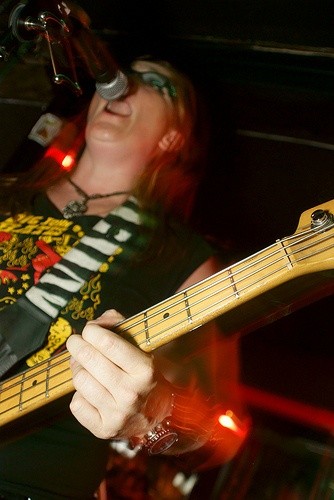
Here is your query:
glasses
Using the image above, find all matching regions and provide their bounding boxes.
[128,65,177,100]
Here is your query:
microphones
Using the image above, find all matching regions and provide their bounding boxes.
[57,2,129,101]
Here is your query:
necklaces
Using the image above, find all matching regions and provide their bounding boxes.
[59,175,138,221]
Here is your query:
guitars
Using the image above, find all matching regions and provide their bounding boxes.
[0,196,334,431]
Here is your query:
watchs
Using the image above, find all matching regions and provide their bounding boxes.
[139,390,182,457]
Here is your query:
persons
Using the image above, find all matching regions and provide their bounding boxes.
[1,53,255,500]
